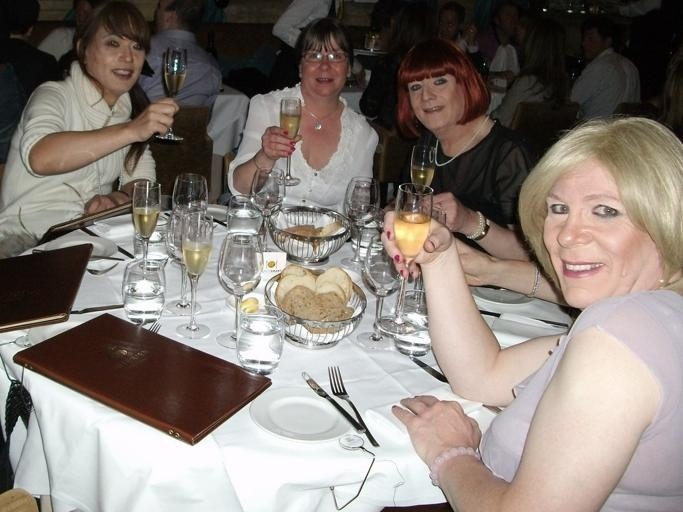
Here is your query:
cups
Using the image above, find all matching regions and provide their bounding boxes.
[165,208,207,315]
[410,144,438,193]
[377,183,435,337]
[393,289,431,358]
[340,177,379,269]
[227,194,264,232]
[235,304,284,376]
[358,235,405,346]
[155,44,189,141]
[364,32,381,51]
[217,234,260,348]
[122,258,165,324]
[175,212,212,339]
[347,205,386,257]
[133,181,161,280]
[172,172,208,208]
[132,216,170,272]
[250,167,285,218]
[279,97,302,186]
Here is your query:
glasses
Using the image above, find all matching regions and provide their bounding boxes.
[302,52,346,63]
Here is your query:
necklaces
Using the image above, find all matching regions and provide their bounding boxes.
[71,81,123,195]
[434,114,489,166]
[303,102,340,131]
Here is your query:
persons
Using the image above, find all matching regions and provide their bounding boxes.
[380,118,683,511]
[0,0,58,96]
[0,60,26,180]
[137,0,222,111]
[569,25,640,123]
[0,0,180,256]
[490,35,562,129]
[435,0,530,73]
[227,18,379,212]
[252,0,345,75]
[455,238,574,307]
[395,38,538,262]
[37,0,99,62]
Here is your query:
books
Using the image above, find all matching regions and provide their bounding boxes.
[39,200,133,241]
[12,313,272,446]
[1,243,93,333]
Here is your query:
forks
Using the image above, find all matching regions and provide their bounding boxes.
[147,320,163,333]
[328,366,388,447]
[86,260,121,276]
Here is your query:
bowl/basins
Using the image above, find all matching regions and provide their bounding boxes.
[263,268,367,349]
[268,205,352,265]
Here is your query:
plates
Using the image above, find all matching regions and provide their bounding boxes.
[224,291,266,317]
[250,385,358,442]
[470,286,532,306]
[45,236,118,257]
[207,200,229,223]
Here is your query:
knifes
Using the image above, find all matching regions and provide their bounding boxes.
[204,215,227,230]
[89,254,125,264]
[70,303,125,314]
[480,311,568,329]
[302,372,369,434]
[79,224,135,259]
[409,355,506,415]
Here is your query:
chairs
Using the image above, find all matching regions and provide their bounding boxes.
[509,100,570,139]
[612,101,660,125]
[371,123,391,179]
[130,107,212,208]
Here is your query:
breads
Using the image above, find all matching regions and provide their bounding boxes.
[317,266,353,299]
[315,281,345,302]
[282,287,313,324]
[279,264,317,281]
[275,274,316,305]
[278,223,342,248]
[309,294,353,335]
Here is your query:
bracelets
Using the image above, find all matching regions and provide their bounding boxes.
[527,263,541,298]
[252,156,273,175]
[467,210,491,242]
[428,447,481,486]
